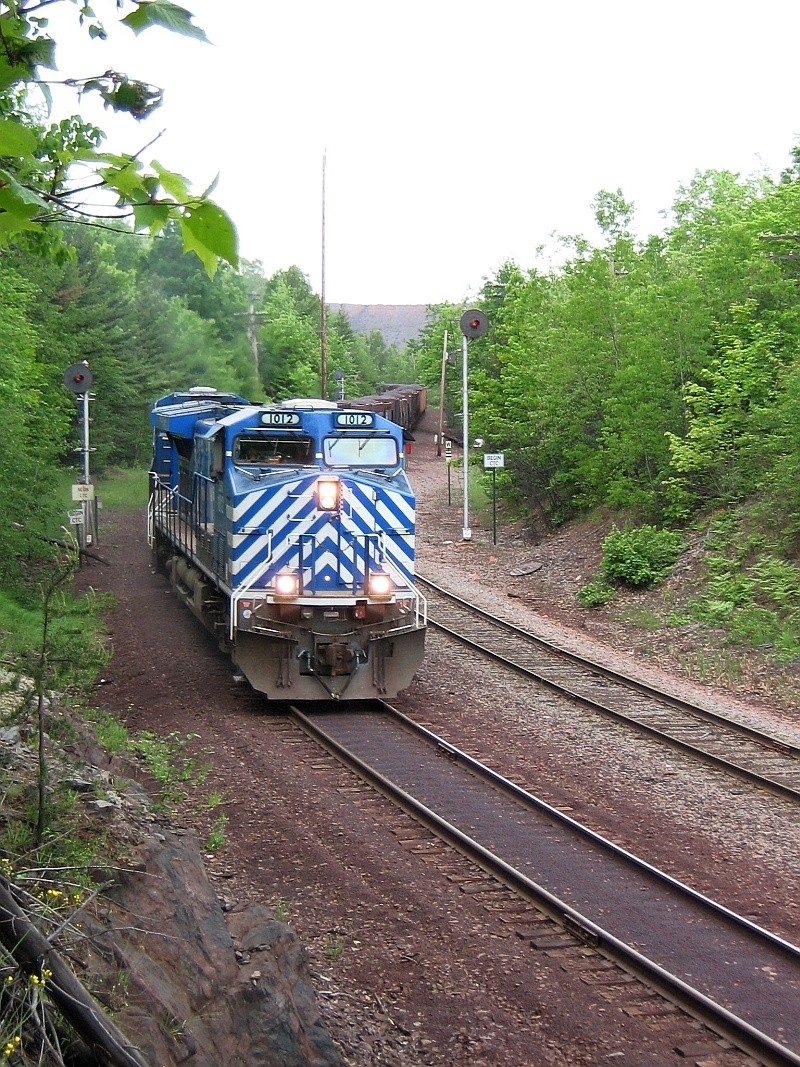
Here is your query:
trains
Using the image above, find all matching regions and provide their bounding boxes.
[146,382,429,700]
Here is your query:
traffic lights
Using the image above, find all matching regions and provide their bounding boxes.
[64,364,94,394]
[460,309,491,339]
